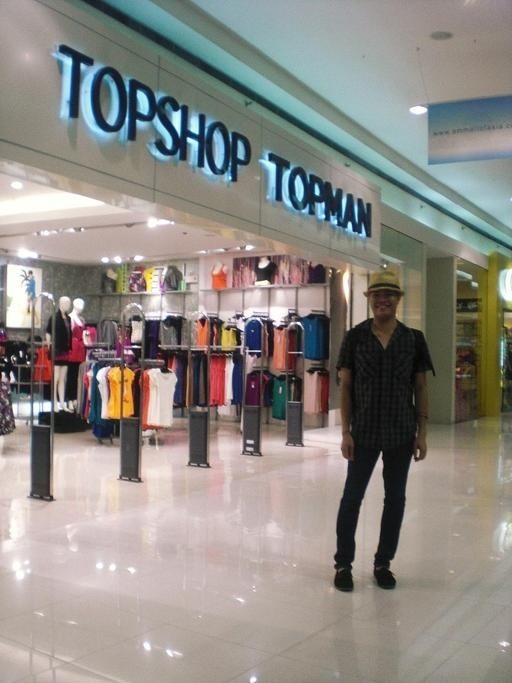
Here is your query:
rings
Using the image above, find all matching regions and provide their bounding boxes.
[340,448,344,450]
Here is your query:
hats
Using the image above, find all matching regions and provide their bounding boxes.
[364,270,404,296]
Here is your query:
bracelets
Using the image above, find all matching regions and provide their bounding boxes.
[341,430,351,434]
[413,408,432,420]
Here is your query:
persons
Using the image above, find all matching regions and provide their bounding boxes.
[254,258,276,288]
[331,269,433,593]
[101,269,118,292]
[46,294,71,416]
[306,259,326,283]
[68,298,89,411]
[209,261,229,290]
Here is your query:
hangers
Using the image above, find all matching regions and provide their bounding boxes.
[84,349,330,383]
[97,309,331,330]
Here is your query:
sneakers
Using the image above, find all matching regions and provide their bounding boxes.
[335,568,353,591]
[374,565,396,588]
